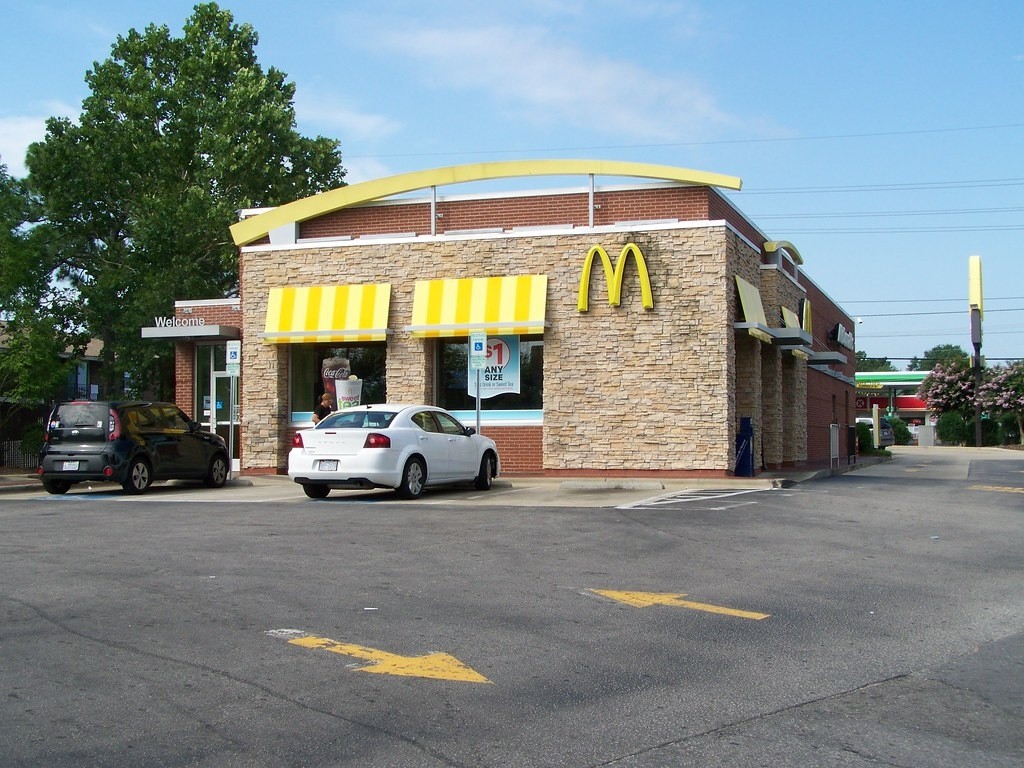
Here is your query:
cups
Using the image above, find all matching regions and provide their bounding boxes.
[322,358,351,412]
[335,379,362,411]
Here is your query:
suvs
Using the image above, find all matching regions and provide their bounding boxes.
[37,400,230,495]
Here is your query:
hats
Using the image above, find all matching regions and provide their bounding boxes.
[322,393,333,399]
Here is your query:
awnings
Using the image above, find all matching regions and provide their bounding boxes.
[406,274,550,337]
[260,284,391,342]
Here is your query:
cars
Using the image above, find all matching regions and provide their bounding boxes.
[287,404,501,501]
[856,418,895,450]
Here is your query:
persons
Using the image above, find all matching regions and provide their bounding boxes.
[311,393,332,427]
